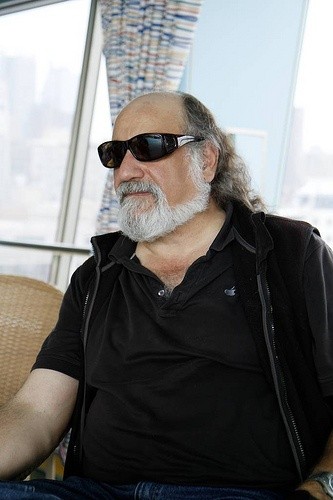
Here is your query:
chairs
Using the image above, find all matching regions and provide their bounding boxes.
[0,273,74,492]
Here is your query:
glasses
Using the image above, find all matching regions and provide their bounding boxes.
[97,133,208,168]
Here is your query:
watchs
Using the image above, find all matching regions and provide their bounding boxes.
[306,471,333,497]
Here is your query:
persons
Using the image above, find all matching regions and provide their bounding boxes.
[0,90,332,500]
